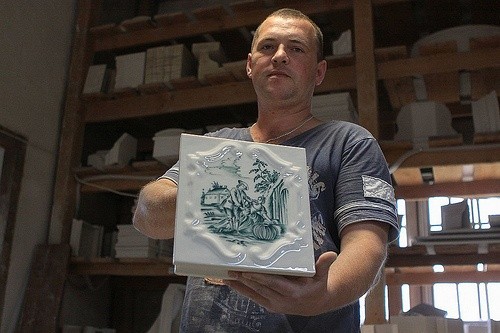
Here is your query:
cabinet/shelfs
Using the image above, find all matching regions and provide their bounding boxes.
[15,0,499,333]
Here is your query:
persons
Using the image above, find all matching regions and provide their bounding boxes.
[132,8,400,333]
